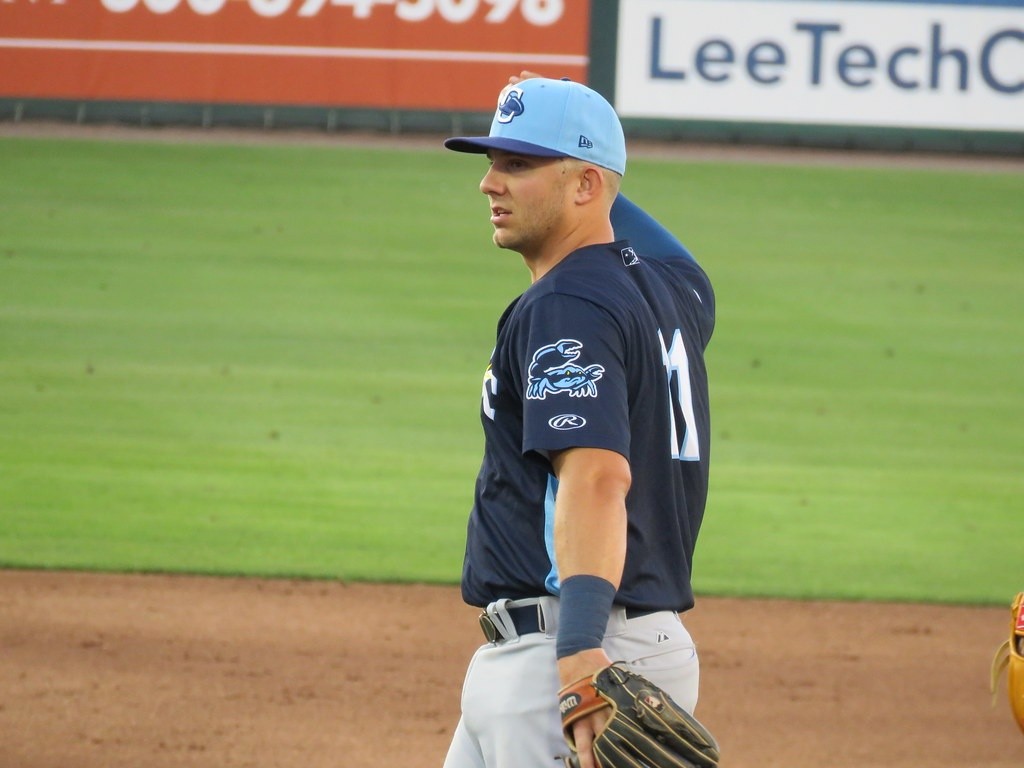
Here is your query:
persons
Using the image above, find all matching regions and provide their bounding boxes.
[444,73,719,768]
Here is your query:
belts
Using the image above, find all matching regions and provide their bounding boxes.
[479,603,657,646]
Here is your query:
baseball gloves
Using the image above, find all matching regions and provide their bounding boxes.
[557,659,720,768]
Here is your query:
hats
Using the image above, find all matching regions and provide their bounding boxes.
[444,77,628,176]
